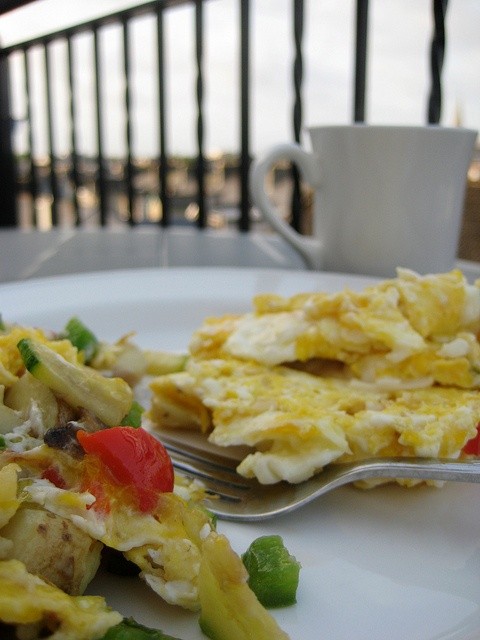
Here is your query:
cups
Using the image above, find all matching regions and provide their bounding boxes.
[247,125,479,278]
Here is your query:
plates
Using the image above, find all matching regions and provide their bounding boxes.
[0,264,480,640]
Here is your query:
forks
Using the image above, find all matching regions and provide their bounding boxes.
[151,432,480,520]
